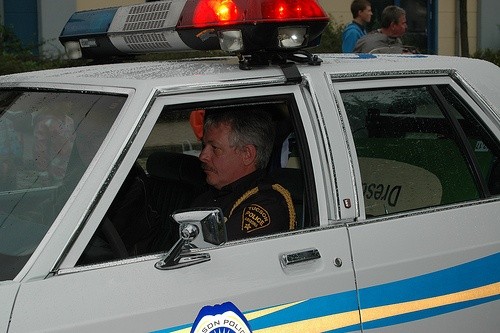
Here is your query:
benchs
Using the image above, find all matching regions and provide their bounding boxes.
[285,128,493,216]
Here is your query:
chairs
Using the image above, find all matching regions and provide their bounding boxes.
[272,168,307,228]
[139,152,209,206]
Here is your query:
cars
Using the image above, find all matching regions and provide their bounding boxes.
[0,0,500,333]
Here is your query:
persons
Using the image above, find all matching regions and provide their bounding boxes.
[341,0,373,53]
[34,103,86,186]
[190,112,296,242]
[17,106,151,246]
[0,118,22,190]
[353,6,413,56]
